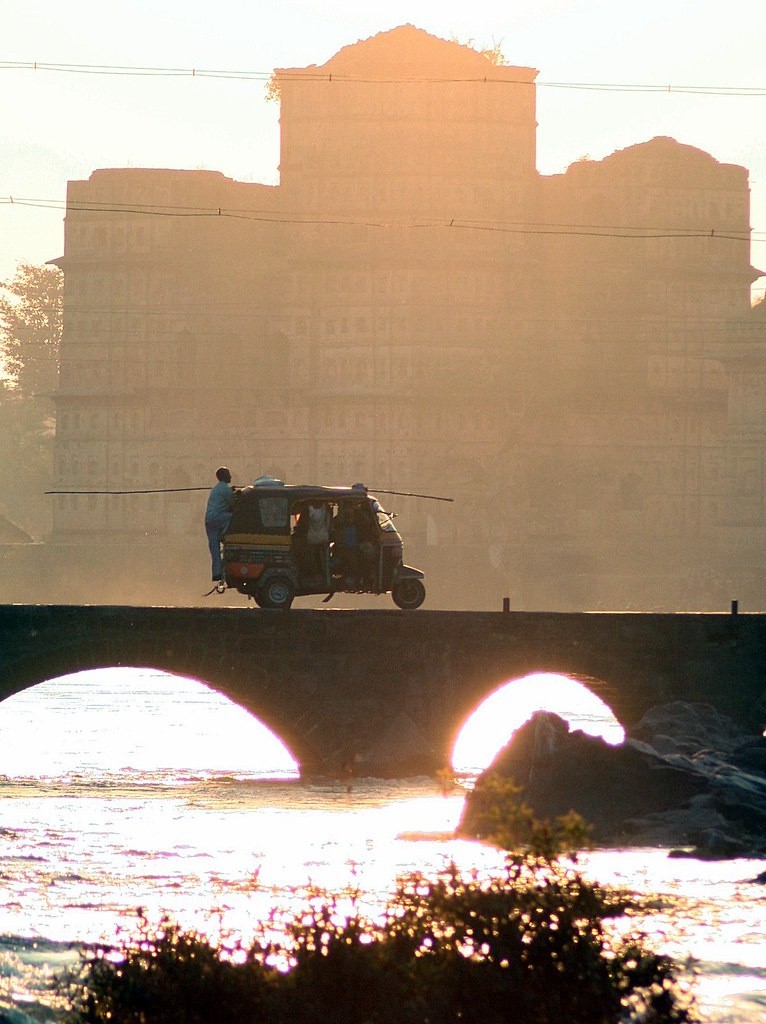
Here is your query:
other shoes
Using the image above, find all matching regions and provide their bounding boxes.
[212,574,222,582]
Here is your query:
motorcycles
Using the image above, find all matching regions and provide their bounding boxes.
[215,478,427,610]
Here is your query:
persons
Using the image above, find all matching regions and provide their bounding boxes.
[295,501,366,587]
[206,467,241,581]
